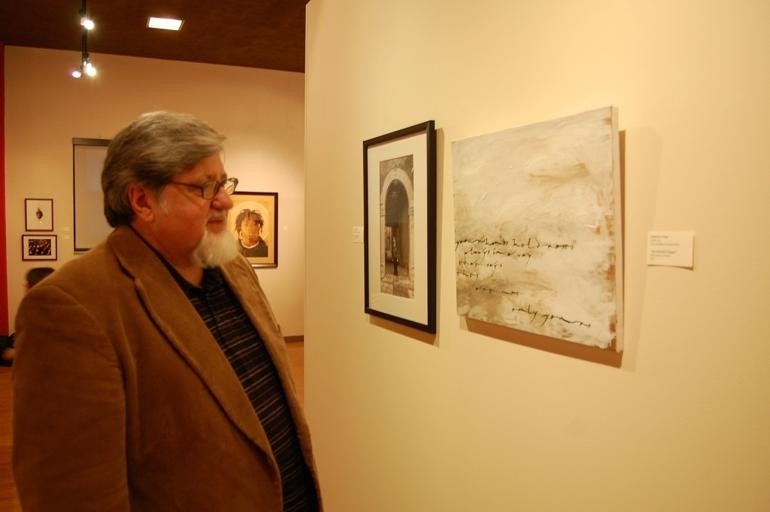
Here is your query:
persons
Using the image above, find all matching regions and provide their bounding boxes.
[232,206,271,258]
[0,265,56,366]
[11,111,328,511]
[389,235,400,275]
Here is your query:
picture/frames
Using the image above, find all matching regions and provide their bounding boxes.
[362,119,438,334]
[25,197,55,232]
[22,234,58,261]
[227,190,279,268]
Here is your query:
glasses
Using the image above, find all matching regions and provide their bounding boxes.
[167,177,239,199]
